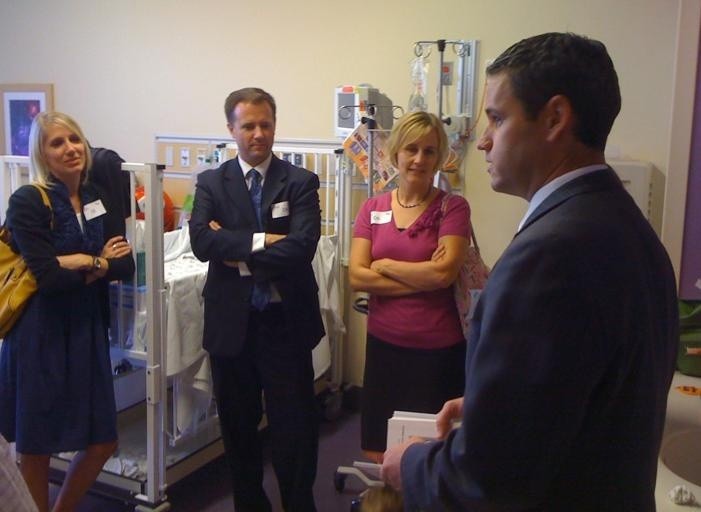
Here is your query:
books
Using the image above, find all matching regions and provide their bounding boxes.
[386,411,439,450]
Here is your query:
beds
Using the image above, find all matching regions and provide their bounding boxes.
[0,134,350,505]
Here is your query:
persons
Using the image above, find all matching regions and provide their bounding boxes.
[189,87,327,512]
[349,110,466,464]
[380,31,678,512]
[0,111,134,512]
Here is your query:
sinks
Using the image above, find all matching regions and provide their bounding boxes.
[661,427,701,486]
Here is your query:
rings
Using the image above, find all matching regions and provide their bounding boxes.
[112,244,116,249]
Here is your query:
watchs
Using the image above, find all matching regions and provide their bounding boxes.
[89,255,100,273]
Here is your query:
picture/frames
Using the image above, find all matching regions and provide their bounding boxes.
[0,84,54,159]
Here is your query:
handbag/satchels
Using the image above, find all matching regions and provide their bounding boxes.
[452,247,490,339]
[0,225,38,339]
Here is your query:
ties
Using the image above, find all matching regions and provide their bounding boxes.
[246,168,271,312]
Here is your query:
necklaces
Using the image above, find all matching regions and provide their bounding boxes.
[396,185,432,208]
